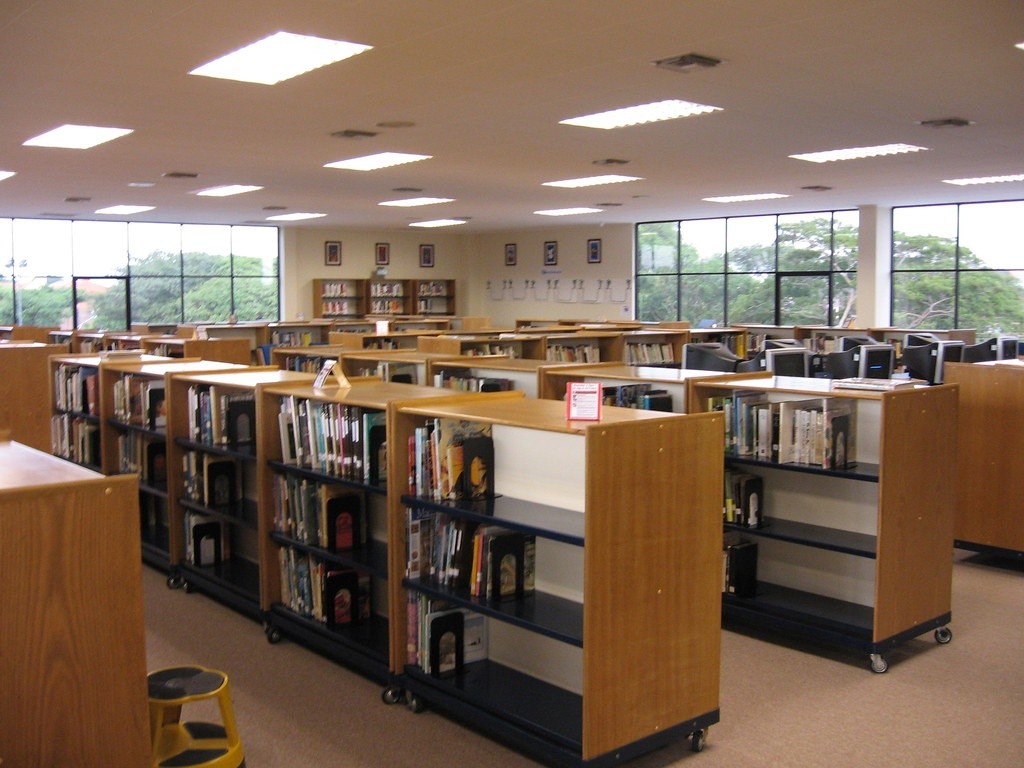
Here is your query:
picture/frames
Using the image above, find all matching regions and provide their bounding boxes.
[587,238,601,263]
[544,240,558,265]
[505,243,517,266]
[325,240,342,265]
[419,243,434,267]
[375,242,390,265]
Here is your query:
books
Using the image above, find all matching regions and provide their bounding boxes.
[692,333,857,602]
[51,336,183,552]
[183,385,252,566]
[270,280,680,412]
[834,378,916,392]
[272,393,387,628]
[404,415,536,676]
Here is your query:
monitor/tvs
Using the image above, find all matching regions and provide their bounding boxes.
[683,331,1024,386]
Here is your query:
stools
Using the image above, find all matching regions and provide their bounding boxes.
[147,663,245,768]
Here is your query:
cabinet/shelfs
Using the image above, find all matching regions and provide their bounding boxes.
[1,340,69,453]
[428,357,587,400]
[98,362,279,578]
[340,350,483,392]
[540,360,738,412]
[944,355,1024,564]
[49,350,201,474]
[1,278,976,363]
[0,425,151,768]
[689,370,960,654]
[258,381,525,704]
[170,370,381,619]
[271,342,416,380]
[394,397,725,768]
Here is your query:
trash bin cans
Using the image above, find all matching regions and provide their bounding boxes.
[146,663,246,768]
